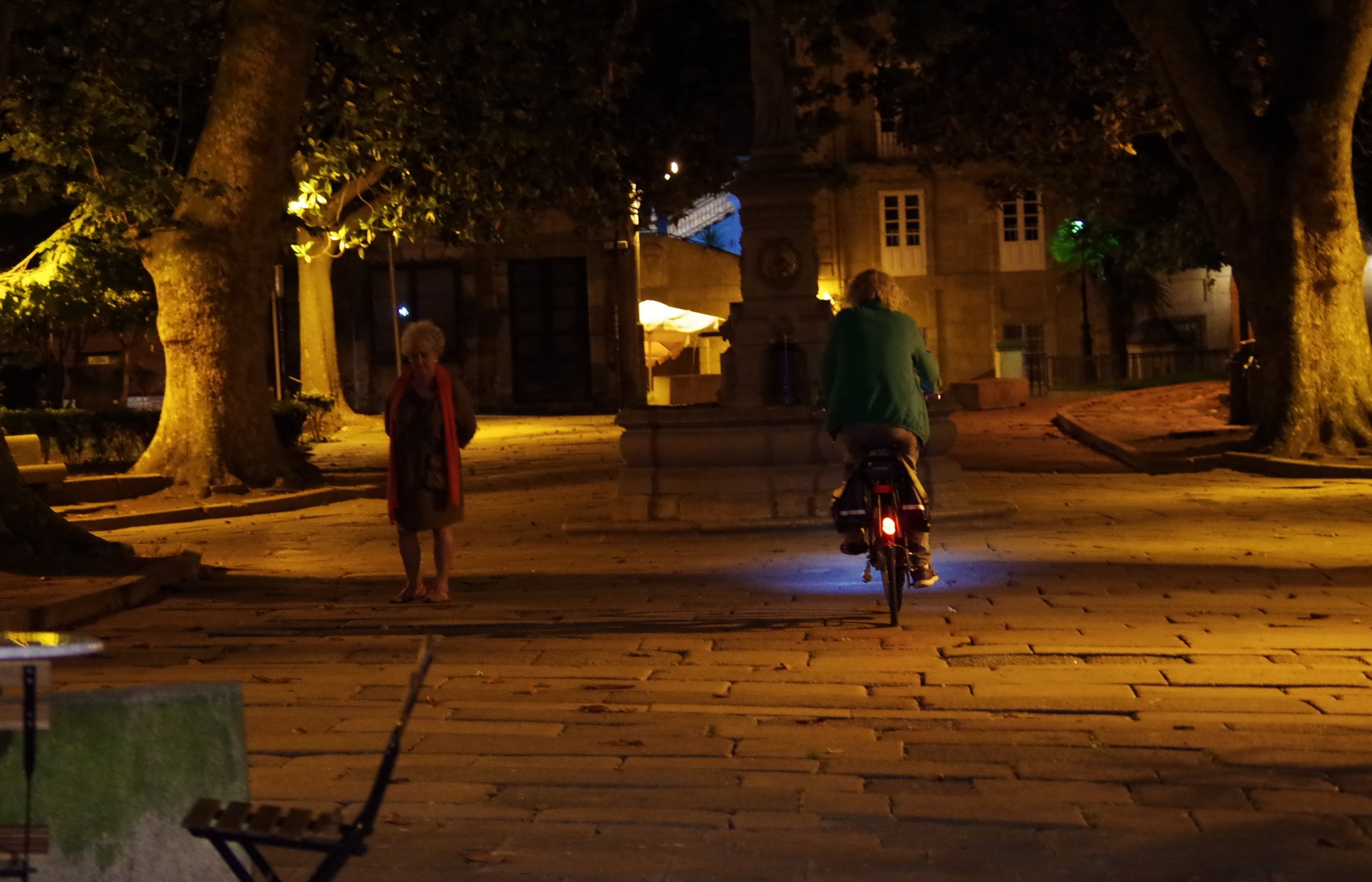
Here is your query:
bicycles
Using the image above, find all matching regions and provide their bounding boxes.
[818,394,942,628]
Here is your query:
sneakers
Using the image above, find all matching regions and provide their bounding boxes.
[912,562,939,588]
[840,528,869,555]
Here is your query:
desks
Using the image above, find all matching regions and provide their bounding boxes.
[0,632,106,882]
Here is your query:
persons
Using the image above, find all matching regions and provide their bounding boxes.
[384,320,476,606]
[820,269,942,586]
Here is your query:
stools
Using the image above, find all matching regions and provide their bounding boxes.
[181,797,374,882]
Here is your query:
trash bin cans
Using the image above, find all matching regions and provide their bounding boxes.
[1225,339,1260,425]
[65,350,131,412]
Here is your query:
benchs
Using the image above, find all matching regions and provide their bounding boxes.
[951,378,1030,410]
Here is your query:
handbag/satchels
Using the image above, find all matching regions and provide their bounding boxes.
[896,458,933,531]
[831,464,874,532]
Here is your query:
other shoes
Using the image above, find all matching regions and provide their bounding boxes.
[422,592,448,602]
[390,587,427,603]
